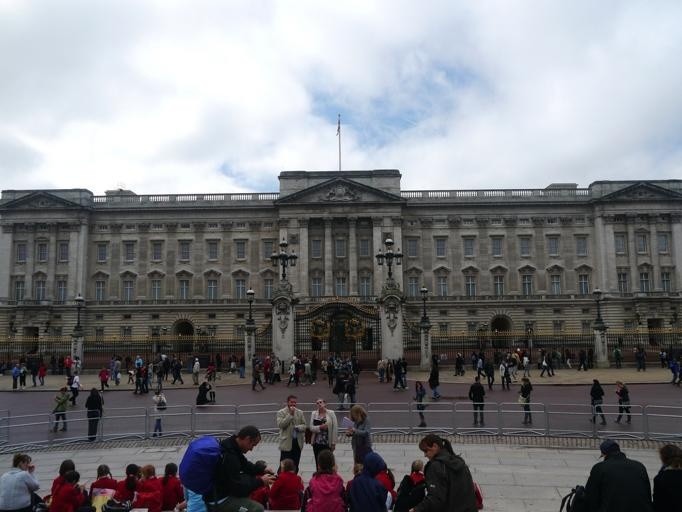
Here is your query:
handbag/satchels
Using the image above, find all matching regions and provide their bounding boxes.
[622,401,632,409]
[157,398,166,410]
[422,395,429,407]
[519,395,527,407]
[594,400,602,405]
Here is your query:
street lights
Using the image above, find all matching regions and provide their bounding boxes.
[73,293,85,335]
[376,237,401,280]
[592,287,605,327]
[418,286,432,327]
[244,287,256,327]
[272,237,297,280]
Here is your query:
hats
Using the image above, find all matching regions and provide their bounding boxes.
[599,440,620,458]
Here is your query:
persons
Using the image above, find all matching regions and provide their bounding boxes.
[321,352,360,410]
[98,353,184,397]
[653,444,681,512]
[539,347,595,377]
[309,398,339,472]
[582,439,652,512]
[151,389,168,437]
[174,426,482,511]
[192,352,245,408]
[48,386,70,433]
[429,354,440,399]
[85,387,105,441]
[468,376,486,426]
[2,453,184,512]
[51,355,81,406]
[518,377,534,424]
[456,349,530,391]
[588,379,607,426]
[252,351,318,392]
[12,361,45,390]
[345,404,374,463]
[412,380,427,427]
[614,346,680,384]
[613,380,631,425]
[377,356,408,391]
[275,394,308,478]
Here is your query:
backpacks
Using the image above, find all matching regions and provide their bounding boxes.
[179,436,234,507]
[394,476,427,512]
[560,486,585,512]
